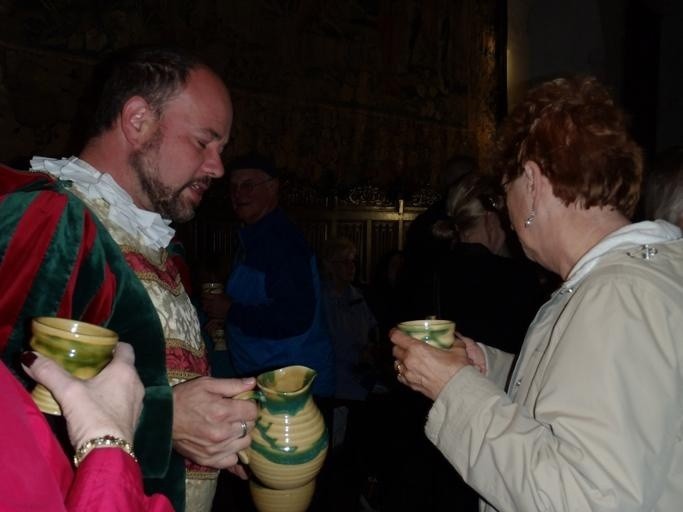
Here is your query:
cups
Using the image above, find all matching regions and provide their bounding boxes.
[248,479,318,511]
[397,318,458,350]
[23,313,120,421]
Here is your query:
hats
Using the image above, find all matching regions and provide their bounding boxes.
[228,151,275,178]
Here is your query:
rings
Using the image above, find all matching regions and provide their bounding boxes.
[238,417,247,441]
[393,360,401,379]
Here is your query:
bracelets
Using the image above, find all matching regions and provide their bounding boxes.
[71,435,138,468]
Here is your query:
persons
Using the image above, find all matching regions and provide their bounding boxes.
[637,142,683,230]
[430,170,558,357]
[200,158,335,510]
[411,156,470,336]
[387,74,683,510]
[366,247,426,319]
[317,234,388,449]
[0,341,175,512]
[0,38,258,511]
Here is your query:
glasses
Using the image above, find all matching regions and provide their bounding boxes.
[229,179,273,193]
[335,259,354,265]
[488,175,519,214]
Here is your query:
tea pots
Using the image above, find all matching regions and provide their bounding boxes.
[228,364,328,489]
